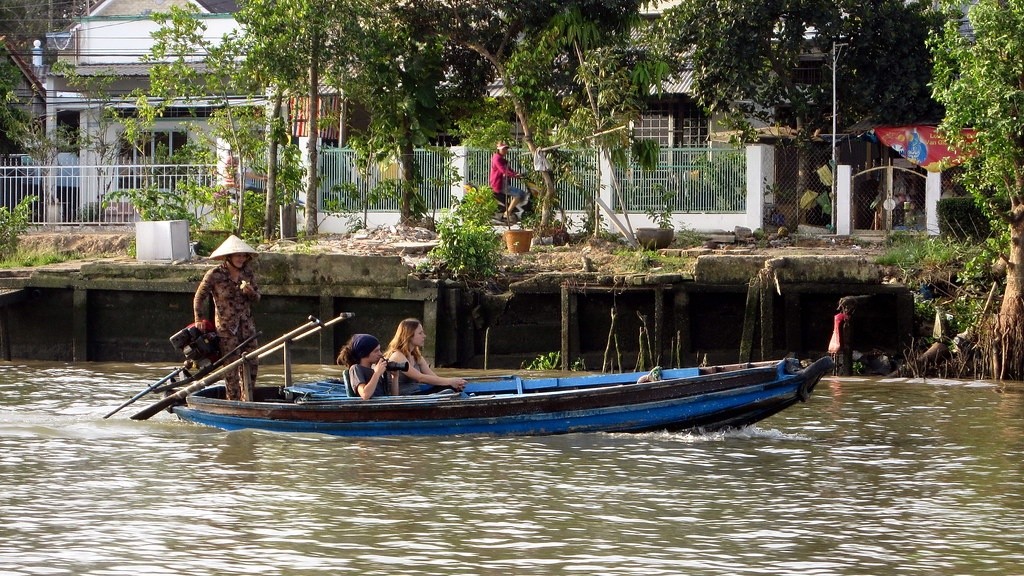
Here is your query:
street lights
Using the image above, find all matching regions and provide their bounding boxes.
[830,41,848,234]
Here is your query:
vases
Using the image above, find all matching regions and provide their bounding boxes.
[638,227,674,248]
[504,229,532,253]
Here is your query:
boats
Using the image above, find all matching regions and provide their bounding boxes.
[156,319,839,438]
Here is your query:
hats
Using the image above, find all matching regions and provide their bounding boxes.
[496,143,508,150]
[209,235,259,260]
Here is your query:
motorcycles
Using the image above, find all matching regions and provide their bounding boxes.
[456,164,564,234]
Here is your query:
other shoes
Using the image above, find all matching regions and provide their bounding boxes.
[502,213,515,219]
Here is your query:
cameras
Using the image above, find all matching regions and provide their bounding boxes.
[382,357,409,372]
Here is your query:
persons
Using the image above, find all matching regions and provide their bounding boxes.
[490,143,525,222]
[338,318,467,401]
[193,235,261,401]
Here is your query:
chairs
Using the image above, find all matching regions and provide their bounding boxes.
[343,369,357,397]
